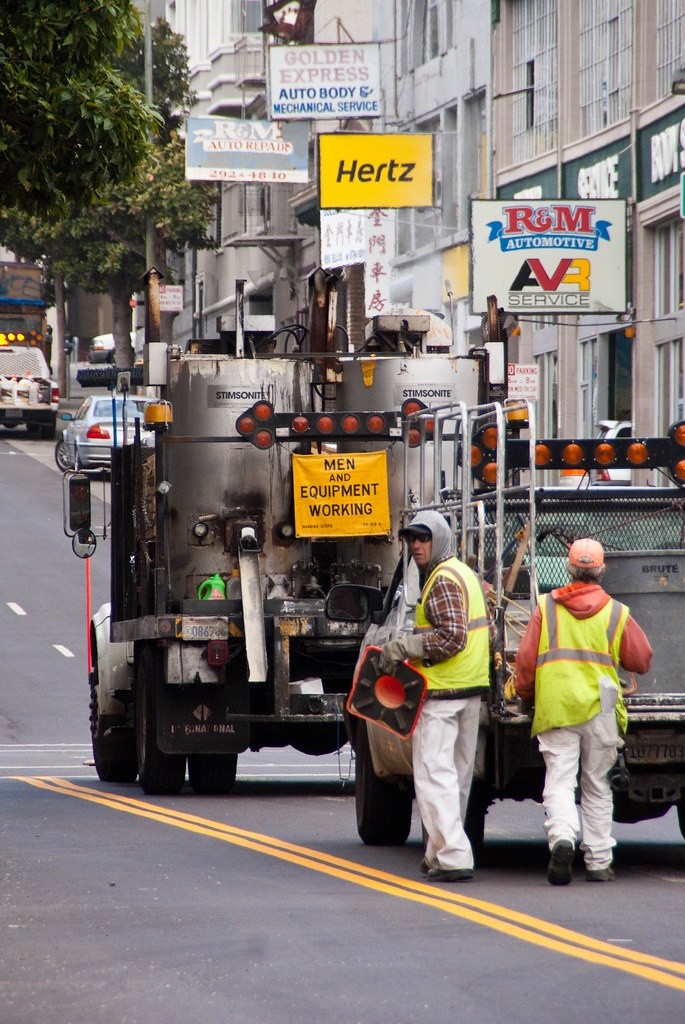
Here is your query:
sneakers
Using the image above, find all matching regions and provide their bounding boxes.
[586,867,616,881]
[547,839,573,885]
[418,858,474,882]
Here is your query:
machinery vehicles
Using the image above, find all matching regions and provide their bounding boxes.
[63,263,531,797]
[325,399,684,866]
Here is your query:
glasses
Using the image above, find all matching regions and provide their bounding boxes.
[404,533,431,543]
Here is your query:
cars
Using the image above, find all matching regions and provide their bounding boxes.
[88,332,136,368]
[588,419,632,488]
[55,394,157,477]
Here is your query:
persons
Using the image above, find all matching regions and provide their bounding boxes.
[514,539,654,884]
[379,510,491,880]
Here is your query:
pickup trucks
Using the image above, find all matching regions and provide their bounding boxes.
[0,345,59,439]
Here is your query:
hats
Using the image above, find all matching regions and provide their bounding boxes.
[569,538,604,568]
[398,525,431,537]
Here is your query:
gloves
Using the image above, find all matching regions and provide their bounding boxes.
[378,633,424,674]
